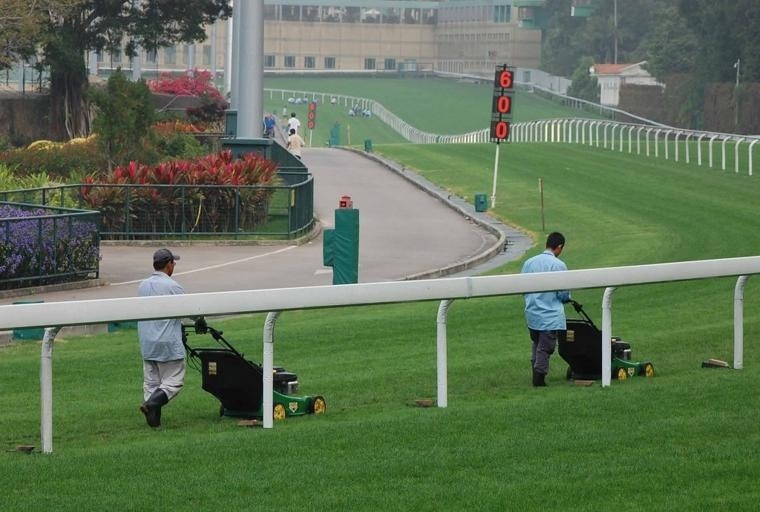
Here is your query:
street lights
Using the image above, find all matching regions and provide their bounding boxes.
[731,58,741,142]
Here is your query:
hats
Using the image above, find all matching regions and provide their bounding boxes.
[153,248,181,261]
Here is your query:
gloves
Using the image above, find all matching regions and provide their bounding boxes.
[194,319,208,334]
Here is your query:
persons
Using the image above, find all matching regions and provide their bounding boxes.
[138,248,207,427]
[288,95,320,104]
[330,96,337,104]
[287,112,300,136]
[518,232,571,388]
[348,102,370,119]
[263,112,275,137]
[288,128,305,159]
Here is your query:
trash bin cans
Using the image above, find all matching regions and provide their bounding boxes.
[12,301,44,340]
[365,139,372,151]
[475,193,487,212]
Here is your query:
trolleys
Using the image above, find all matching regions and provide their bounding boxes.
[180,321,331,421]
[557,299,658,387]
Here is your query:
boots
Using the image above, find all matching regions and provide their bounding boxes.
[533,370,549,386]
[140,387,168,426]
